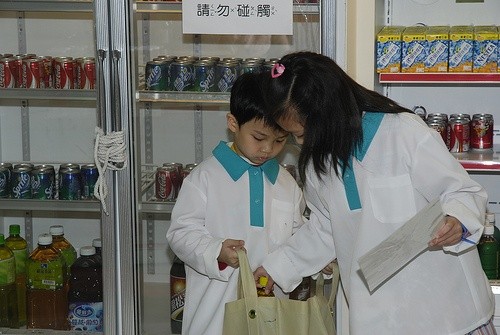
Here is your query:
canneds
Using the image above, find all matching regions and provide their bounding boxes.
[0,162,100,201]
[0,53,97,90]
[145,56,281,93]
[428,113,494,153]
[155,163,197,202]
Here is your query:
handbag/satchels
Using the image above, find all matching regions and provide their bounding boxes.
[222,246,342,335]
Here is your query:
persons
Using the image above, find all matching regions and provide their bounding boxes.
[268,52,497,335]
[166,72,337,335]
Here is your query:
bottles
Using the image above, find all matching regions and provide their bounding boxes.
[5,225,28,327]
[0,234,19,328]
[26,234,69,330]
[476,212,500,281]
[93,240,101,254]
[49,226,77,276]
[68,247,103,332]
[256,275,275,298]
[170,255,186,334]
